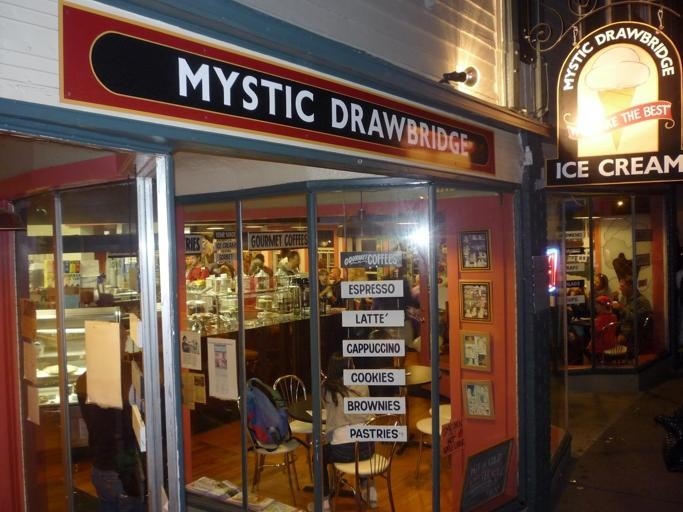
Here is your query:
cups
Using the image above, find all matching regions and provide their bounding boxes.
[326,305,331,313]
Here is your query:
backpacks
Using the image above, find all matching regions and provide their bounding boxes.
[240,379,291,451]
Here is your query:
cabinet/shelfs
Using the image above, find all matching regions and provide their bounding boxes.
[33,305,121,452]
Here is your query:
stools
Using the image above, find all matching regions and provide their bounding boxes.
[414,403,450,479]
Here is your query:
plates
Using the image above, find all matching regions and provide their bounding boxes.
[45,364,78,376]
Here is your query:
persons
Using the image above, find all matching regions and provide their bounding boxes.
[184,248,344,308]
[562,273,651,365]
[306,351,378,512]
[74,320,146,512]
[370,257,420,346]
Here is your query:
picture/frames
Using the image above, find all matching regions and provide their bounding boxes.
[454,225,496,422]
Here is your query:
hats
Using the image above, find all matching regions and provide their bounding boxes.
[594,295,612,306]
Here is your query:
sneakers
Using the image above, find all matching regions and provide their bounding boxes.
[307,500,329,512]
[361,488,376,507]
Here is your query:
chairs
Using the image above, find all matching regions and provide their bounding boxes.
[595,320,633,365]
[236,374,401,511]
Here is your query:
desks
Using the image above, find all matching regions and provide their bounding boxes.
[378,365,441,456]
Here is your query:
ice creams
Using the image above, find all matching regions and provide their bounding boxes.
[584,47,651,149]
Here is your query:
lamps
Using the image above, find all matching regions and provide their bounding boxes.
[335,191,384,240]
[438,66,478,88]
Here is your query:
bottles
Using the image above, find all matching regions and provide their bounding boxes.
[205,272,233,297]
[254,268,289,293]
[320,295,326,315]
